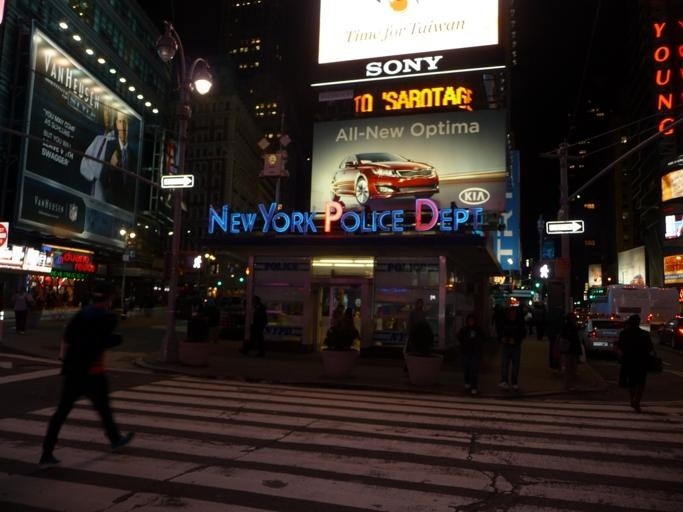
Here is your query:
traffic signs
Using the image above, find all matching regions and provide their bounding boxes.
[545,220,585,235]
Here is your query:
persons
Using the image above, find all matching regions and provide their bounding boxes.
[491,302,547,341]
[332,303,347,326]
[10,284,69,336]
[99,111,136,213]
[238,295,268,358]
[455,313,487,395]
[618,320,633,388]
[497,306,523,391]
[78,101,117,203]
[622,314,655,413]
[560,330,583,392]
[38,285,135,471]
[334,306,354,349]
[402,298,425,377]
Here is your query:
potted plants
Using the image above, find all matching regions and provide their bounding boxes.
[176,316,208,366]
[319,323,360,378]
[404,319,443,386]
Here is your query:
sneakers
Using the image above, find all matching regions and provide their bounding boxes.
[464,382,518,394]
[629,399,643,414]
[38,457,64,470]
[110,431,134,450]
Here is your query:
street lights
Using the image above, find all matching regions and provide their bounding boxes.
[119,226,136,315]
[155,20,215,365]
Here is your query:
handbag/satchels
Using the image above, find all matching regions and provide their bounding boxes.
[646,356,662,373]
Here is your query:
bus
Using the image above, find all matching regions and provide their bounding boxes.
[589,286,650,325]
[646,287,682,330]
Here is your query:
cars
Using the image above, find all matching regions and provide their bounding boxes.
[329,150,439,206]
[581,318,626,359]
[656,312,683,349]
